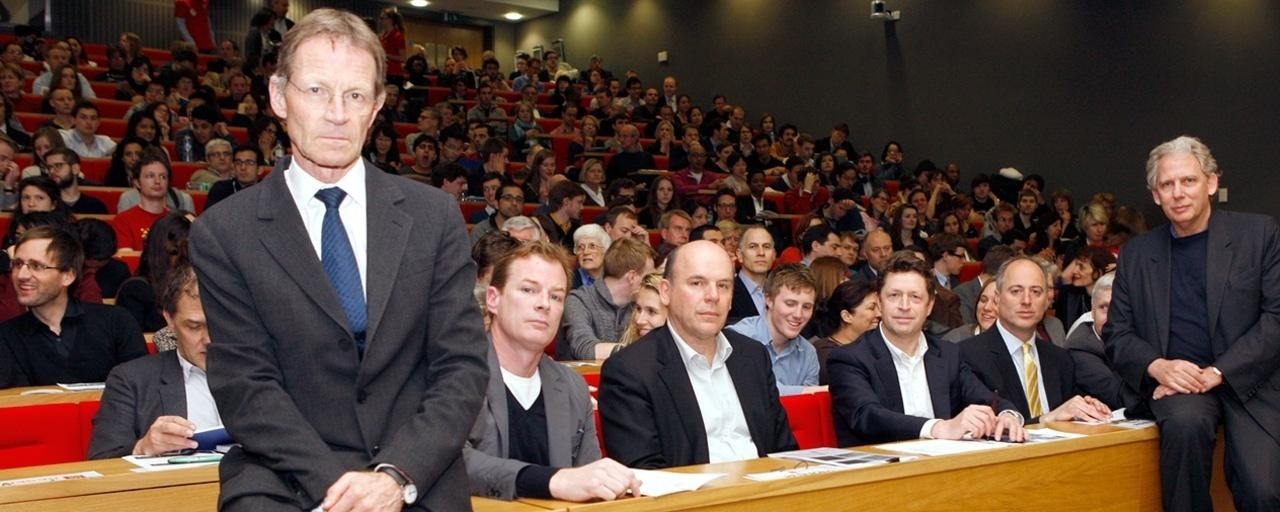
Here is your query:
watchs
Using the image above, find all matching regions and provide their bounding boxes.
[1000,410,1020,423]
[1212,368,1222,376]
[380,461,420,504]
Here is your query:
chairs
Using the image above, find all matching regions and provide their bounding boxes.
[383,38,1131,265]
[593,408,608,461]
[584,373,601,399]
[817,389,837,449]
[0,403,80,471]
[1,31,269,175]
[780,393,822,450]
[78,401,108,462]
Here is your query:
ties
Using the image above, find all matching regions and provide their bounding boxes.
[1021,343,1044,419]
[315,187,366,362]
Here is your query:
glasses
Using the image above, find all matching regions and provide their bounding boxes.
[576,243,604,251]
[235,159,258,166]
[584,124,597,128]
[443,144,464,151]
[10,258,61,270]
[110,57,123,61]
[208,151,232,157]
[618,194,635,199]
[266,127,275,135]
[950,252,965,258]
[887,150,897,154]
[134,67,148,72]
[420,115,432,120]
[500,194,524,201]
[6,48,22,56]
[718,202,735,209]
[46,161,70,169]
[690,152,705,157]
[278,77,377,105]
[147,87,164,96]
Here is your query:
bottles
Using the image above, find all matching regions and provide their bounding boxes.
[274,139,284,166]
[182,132,194,163]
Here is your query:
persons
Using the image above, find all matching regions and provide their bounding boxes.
[842,233,860,265]
[853,230,896,286]
[931,242,968,286]
[728,230,779,323]
[1057,246,1111,334]
[459,241,643,501]
[1064,273,1122,402]
[1030,257,1067,349]
[1102,140,1280,512]
[826,252,1029,442]
[802,225,841,271]
[954,245,1016,322]
[739,264,823,388]
[86,265,234,460]
[193,11,491,512]
[598,240,800,461]
[618,271,680,359]
[1003,231,1030,257]
[781,214,825,263]
[564,243,648,360]
[0,227,149,389]
[811,281,880,371]
[807,257,848,298]
[690,226,725,251]
[573,225,611,288]
[958,257,1114,426]
[942,281,1003,341]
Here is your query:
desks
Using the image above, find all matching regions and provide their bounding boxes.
[72,184,210,197]
[118,248,140,256]
[0,451,230,512]
[0,208,128,223]
[466,414,1238,512]
[1,383,107,409]
[557,360,606,374]
